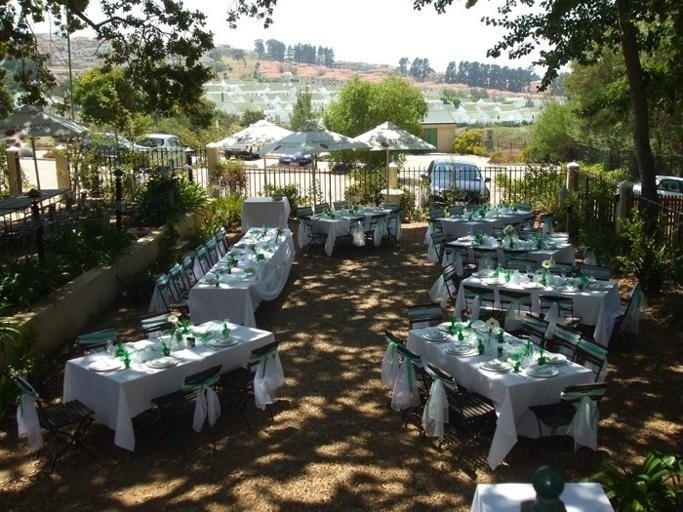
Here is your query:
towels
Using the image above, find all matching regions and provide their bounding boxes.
[151,356,177,367]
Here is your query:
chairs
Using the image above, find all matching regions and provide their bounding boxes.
[78,311,118,352]
[408,303,441,329]
[422,361,495,462]
[138,201,403,343]
[219,341,285,429]
[528,382,611,466]
[424,202,645,382]
[381,328,430,428]
[10,368,95,475]
[150,364,223,460]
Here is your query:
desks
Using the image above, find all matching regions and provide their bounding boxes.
[241,196,291,233]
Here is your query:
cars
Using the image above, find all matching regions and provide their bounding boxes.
[615,170,683,203]
[127,128,189,154]
[419,157,494,212]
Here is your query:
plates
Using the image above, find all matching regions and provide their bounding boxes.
[422,321,568,378]
[471,268,597,293]
[457,235,562,251]
[88,324,238,372]
[199,229,285,284]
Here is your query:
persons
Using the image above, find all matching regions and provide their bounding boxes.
[213,120,296,198]
[0,103,87,206]
[256,118,373,219]
[349,120,437,203]
